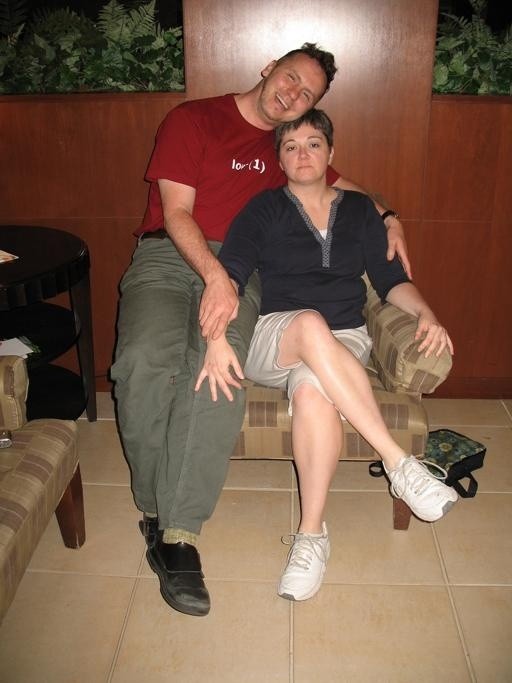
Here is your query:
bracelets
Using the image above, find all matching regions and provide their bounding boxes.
[379,209,401,225]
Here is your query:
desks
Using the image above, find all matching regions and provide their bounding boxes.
[2,220,101,424]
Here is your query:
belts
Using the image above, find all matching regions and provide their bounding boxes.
[140,229,170,239]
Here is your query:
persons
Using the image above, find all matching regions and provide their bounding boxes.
[188,107,459,601]
[107,43,411,620]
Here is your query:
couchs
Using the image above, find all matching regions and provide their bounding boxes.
[229,257,458,538]
[0,351,84,628]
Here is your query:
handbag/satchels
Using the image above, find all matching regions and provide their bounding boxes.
[423,429,486,498]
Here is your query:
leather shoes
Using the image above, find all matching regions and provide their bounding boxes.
[137,512,211,616]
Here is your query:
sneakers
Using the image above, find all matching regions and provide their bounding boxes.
[277,522,330,601]
[382,457,459,522]
